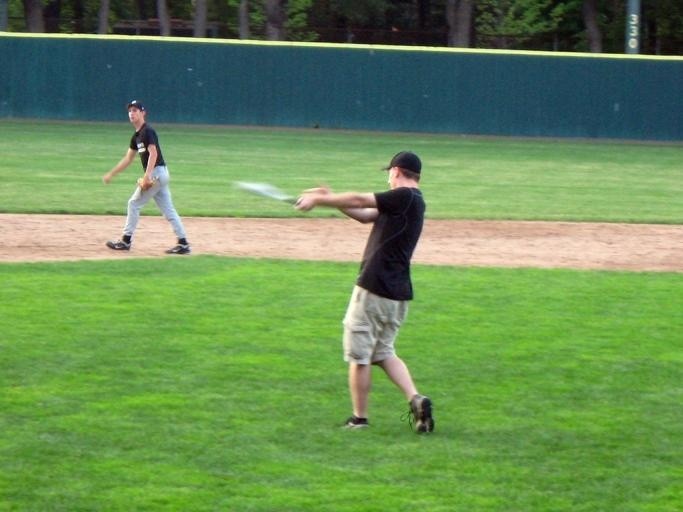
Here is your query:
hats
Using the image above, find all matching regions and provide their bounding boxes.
[381,152,422,174]
[128,101,145,111]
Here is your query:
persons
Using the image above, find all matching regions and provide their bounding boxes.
[295,152,435,432]
[103,100,191,255]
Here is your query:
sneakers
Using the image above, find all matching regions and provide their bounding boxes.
[409,394,434,434]
[166,244,191,254]
[343,416,368,428]
[107,240,131,250]
